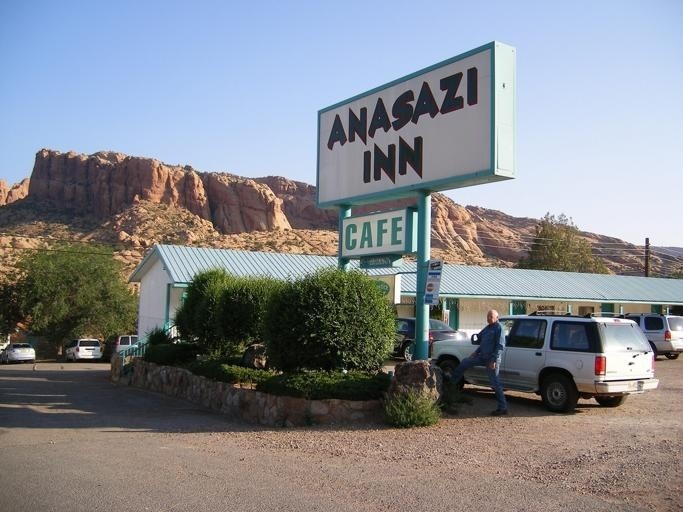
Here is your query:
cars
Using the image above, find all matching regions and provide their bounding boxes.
[0,342,10,354]
[64,338,105,361]
[383,316,469,364]
[0,343,38,364]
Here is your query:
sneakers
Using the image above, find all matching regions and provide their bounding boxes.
[492,408,507,416]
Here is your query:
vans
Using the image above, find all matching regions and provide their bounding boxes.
[106,335,138,360]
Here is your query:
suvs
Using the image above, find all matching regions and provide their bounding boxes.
[429,308,660,413]
[611,311,682,363]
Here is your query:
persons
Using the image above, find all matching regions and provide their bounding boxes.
[444,308,508,416]
